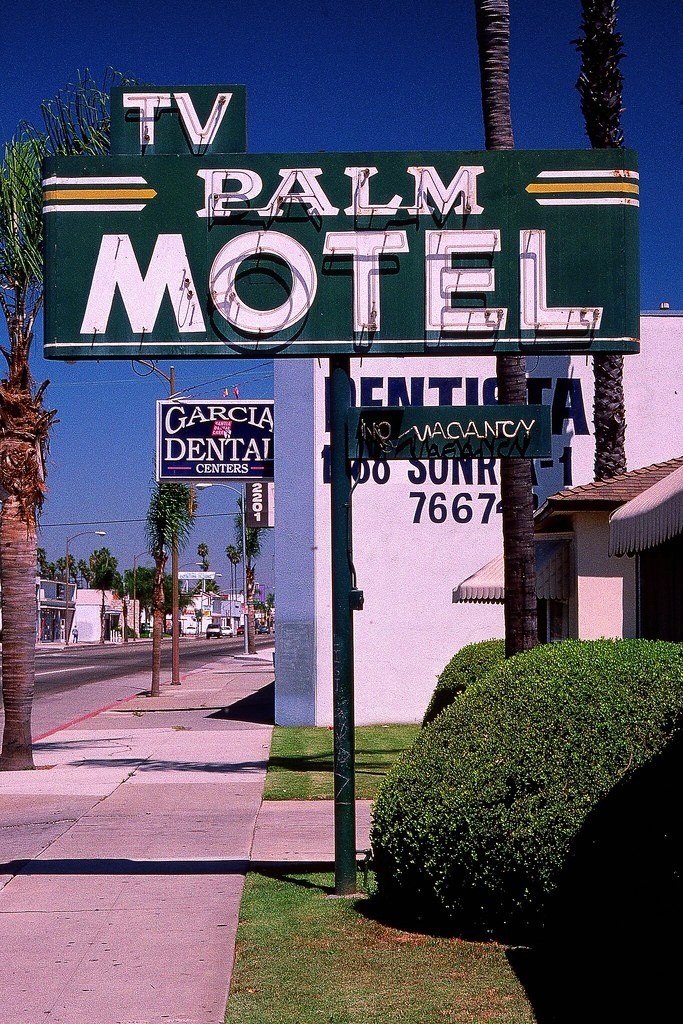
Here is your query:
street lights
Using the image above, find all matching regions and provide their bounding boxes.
[65,531,106,645]
[172,562,204,638]
[197,573,222,634]
[133,549,165,641]
[197,482,253,659]
